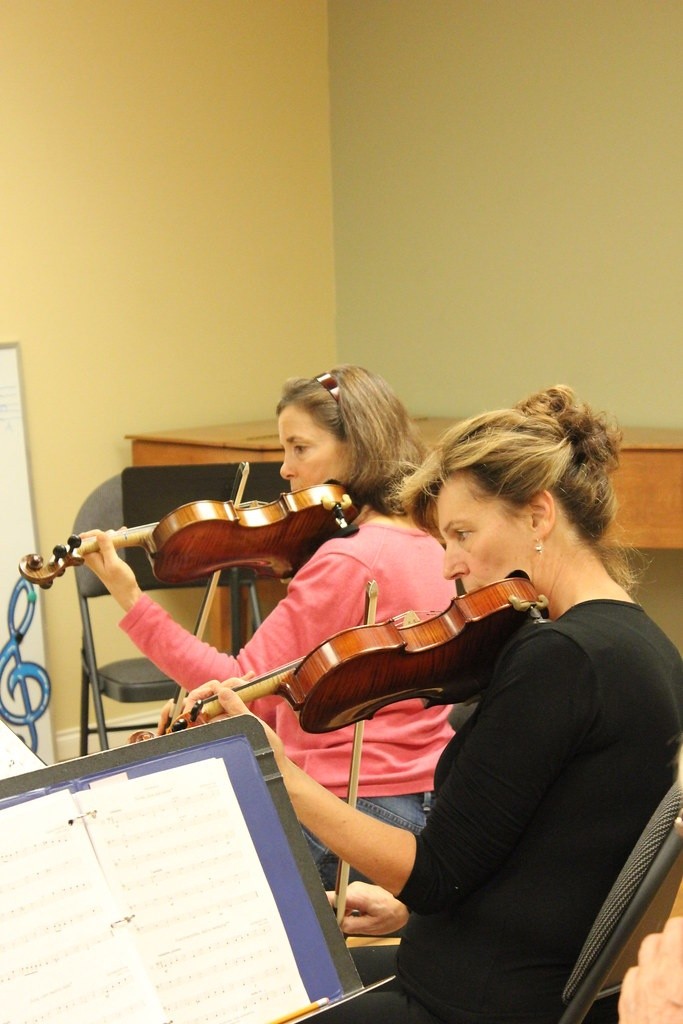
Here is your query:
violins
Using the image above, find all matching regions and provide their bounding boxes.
[18,482,354,589]
[130,575,549,749]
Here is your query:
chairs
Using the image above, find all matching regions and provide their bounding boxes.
[69,472,263,758]
[554,778,683,1024]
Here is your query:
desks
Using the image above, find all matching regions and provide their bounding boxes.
[127,416,683,656]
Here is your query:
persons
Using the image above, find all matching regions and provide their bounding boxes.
[74,365,457,892]
[157,386,683,1024]
